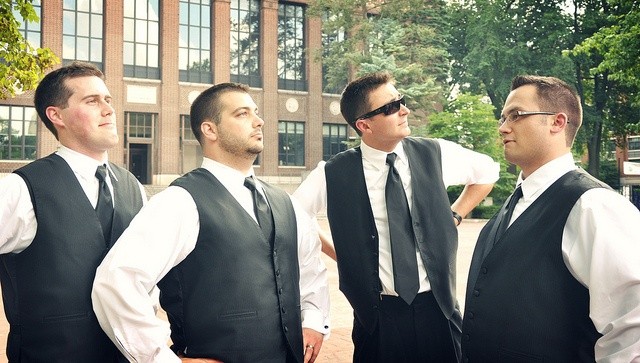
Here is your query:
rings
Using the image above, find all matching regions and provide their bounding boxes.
[306,345,314,349]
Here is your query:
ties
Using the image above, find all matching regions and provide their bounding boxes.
[243,177,275,245]
[95,165,114,251]
[481,186,523,267]
[385,152,420,306]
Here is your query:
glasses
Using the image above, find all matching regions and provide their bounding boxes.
[498,110,570,126]
[354,95,405,126]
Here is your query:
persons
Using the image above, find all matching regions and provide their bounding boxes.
[461,75,640,363]
[0,62,161,363]
[292,73,500,363]
[91,83,330,363]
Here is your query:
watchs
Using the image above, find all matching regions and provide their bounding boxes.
[451,210,462,226]
